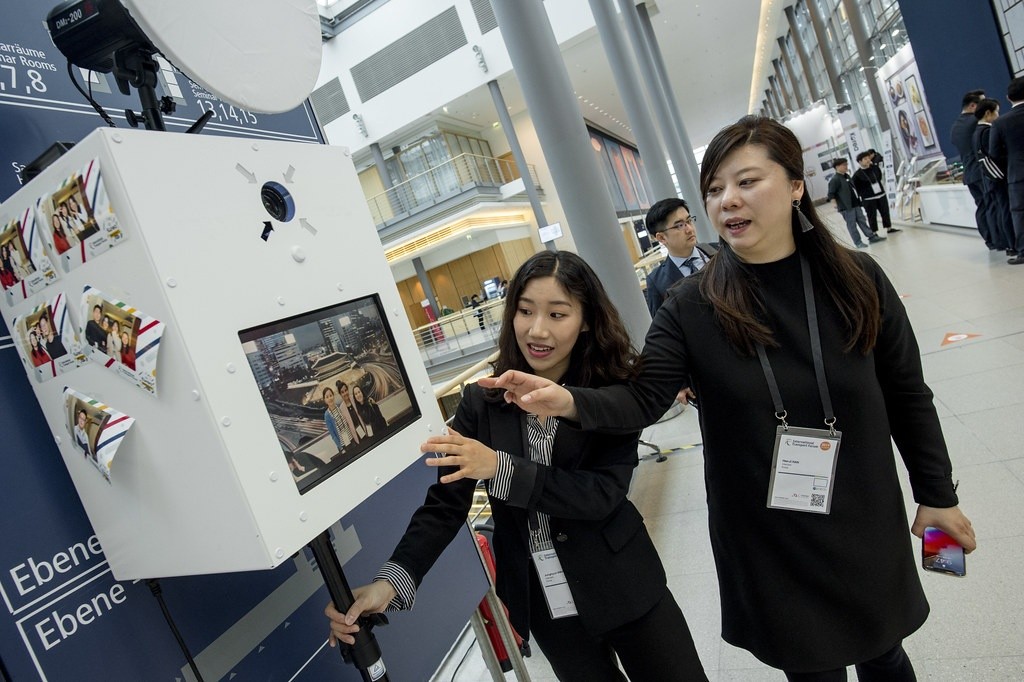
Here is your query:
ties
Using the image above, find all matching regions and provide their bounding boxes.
[682,258,698,275]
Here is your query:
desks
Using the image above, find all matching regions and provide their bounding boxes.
[915,181,978,229]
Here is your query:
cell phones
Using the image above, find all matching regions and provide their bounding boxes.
[921,526,966,576]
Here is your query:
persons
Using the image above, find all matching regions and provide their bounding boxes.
[86,304,136,372]
[323,379,388,452]
[324,250,710,682]
[501,280,508,299]
[471,294,485,330]
[644,197,719,411]
[0,239,31,291]
[25,316,68,367]
[826,149,903,248]
[478,114,977,682]
[73,409,93,458]
[52,194,100,254]
[950,76,1024,264]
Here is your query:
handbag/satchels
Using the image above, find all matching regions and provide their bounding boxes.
[473,310,478,317]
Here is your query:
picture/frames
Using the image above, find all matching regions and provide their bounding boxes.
[905,75,935,149]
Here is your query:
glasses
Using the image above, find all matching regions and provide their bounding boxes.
[655,216,696,230]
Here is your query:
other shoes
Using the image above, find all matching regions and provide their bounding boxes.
[1007,256,1024,265]
[857,242,868,248]
[887,228,900,234]
[1006,248,1018,256]
[868,234,886,244]
